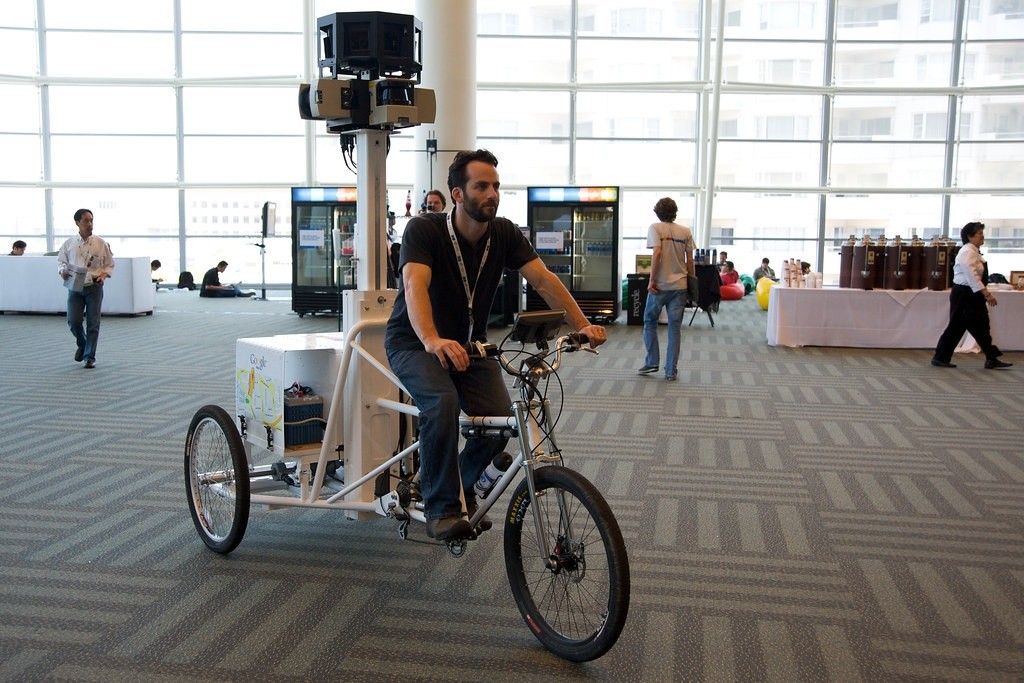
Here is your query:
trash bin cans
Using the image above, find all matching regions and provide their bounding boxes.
[627,272,649,325]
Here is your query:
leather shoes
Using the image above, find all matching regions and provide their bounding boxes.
[984,359,1012,370]
[931,356,957,368]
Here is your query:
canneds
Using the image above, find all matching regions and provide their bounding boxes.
[536,230,573,255]
[694,255,704,265]
[341,224,353,233]
[546,265,571,273]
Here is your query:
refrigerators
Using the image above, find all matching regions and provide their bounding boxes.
[290,185,363,319]
[525,184,623,324]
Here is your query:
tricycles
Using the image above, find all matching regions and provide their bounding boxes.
[184,312,629,661]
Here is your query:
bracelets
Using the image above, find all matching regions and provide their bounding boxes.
[986,293,990,297]
[579,325,588,330]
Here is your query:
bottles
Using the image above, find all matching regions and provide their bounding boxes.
[587,241,614,255]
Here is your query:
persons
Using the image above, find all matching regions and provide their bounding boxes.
[385,149,606,538]
[9,241,26,255]
[200,261,256,297]
[151,260,164,281]
[752,258,775,284]
[637,198,697,380]
[715,251,738,283]
[931,222,1014,369]
[419,190,446,214]
[391,243,401,278]
[57,209,115,368]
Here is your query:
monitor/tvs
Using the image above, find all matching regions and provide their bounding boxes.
[262,201,276,238]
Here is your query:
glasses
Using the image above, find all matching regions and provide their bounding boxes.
[87,255,94,267]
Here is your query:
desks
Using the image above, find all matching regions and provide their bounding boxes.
[765,287,1024,351]
[0,256,155,318]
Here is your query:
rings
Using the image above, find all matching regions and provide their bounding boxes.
[599,331,602,333]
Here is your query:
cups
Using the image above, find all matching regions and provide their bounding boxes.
[693,248,717,266]
[780,258,823,289]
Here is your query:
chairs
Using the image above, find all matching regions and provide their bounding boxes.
[738,272,755,294]
[719,279,745,299]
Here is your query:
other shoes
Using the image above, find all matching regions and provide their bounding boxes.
[84,360,96,369]
[74,349,85,361]
[468,505,492,531]
[427,516,475,543]
[666,369,678,381]
[639,365,659,374]
